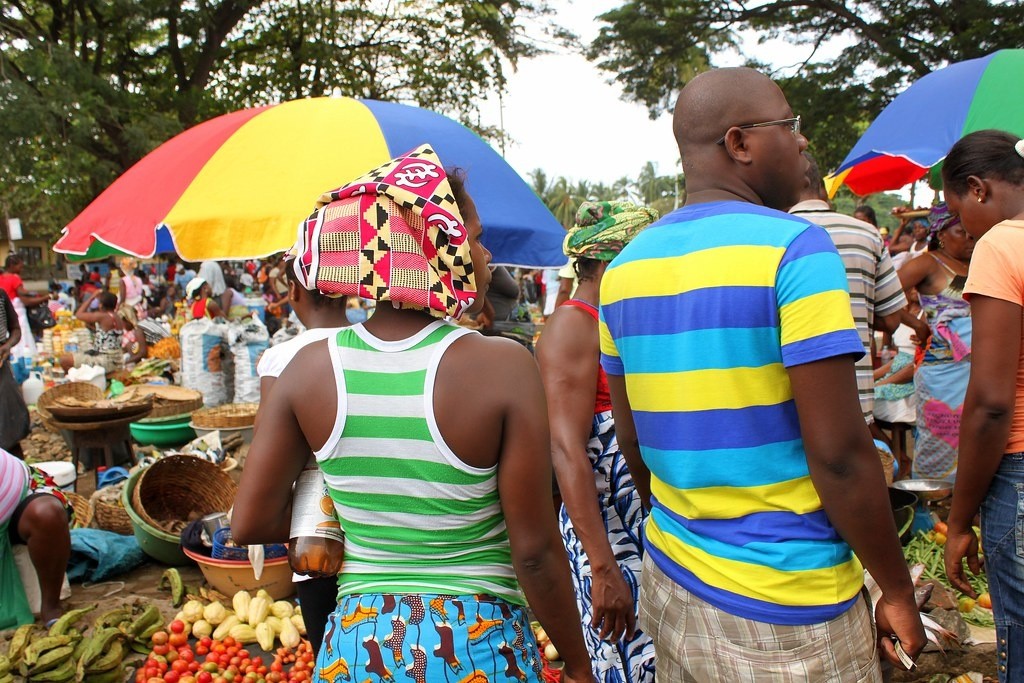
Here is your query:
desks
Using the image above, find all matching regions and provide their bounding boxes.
[48,408,155,494]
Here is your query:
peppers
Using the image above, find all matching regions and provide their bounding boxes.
[538,638,566,683]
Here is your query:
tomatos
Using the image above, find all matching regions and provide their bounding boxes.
[135,620,314,683]
[927,523,992,612]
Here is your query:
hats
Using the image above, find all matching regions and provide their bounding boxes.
[240,273,254,288]
[186,277,206,300]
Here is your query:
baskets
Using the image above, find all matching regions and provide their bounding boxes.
[37,382,104,422]
[94,497,134,536]
[62,491,92,529]
[190,403,259,427]
[119,384,203,419]
[212,527,287,561]
[131,454,240,538]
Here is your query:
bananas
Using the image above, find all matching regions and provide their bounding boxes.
[0,568,184,683]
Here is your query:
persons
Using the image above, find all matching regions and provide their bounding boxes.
[597,68,927,683]
[899,199,978,480]
[230,145,594,683]
[945,129,1024,683]
[0,448,71,629]
[532,199,660,683]
[0,249,1024,683]
[786,152,911,477]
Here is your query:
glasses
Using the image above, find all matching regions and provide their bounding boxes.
[716,115,802,144]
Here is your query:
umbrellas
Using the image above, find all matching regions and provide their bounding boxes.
[57,87,569,270]
[818,49,1023,202]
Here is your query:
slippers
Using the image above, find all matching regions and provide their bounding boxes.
[41,618,80,631]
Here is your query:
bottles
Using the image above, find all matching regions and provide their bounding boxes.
[110,379,125,397]
[21,370,45,406]
[881,346,891,366]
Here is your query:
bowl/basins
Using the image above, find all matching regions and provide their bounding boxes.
[129,456,238,475]
[129,403,206,449]
[121,465,193,564]
[188,421,255,445]
[875,349,897,369]
[182,546,296,601]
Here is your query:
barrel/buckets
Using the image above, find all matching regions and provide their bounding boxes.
[888,487,919,547]
[26,462,76,508]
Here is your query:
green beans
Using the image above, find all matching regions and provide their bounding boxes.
[963,606,995,627]
[898,505,988,597]
